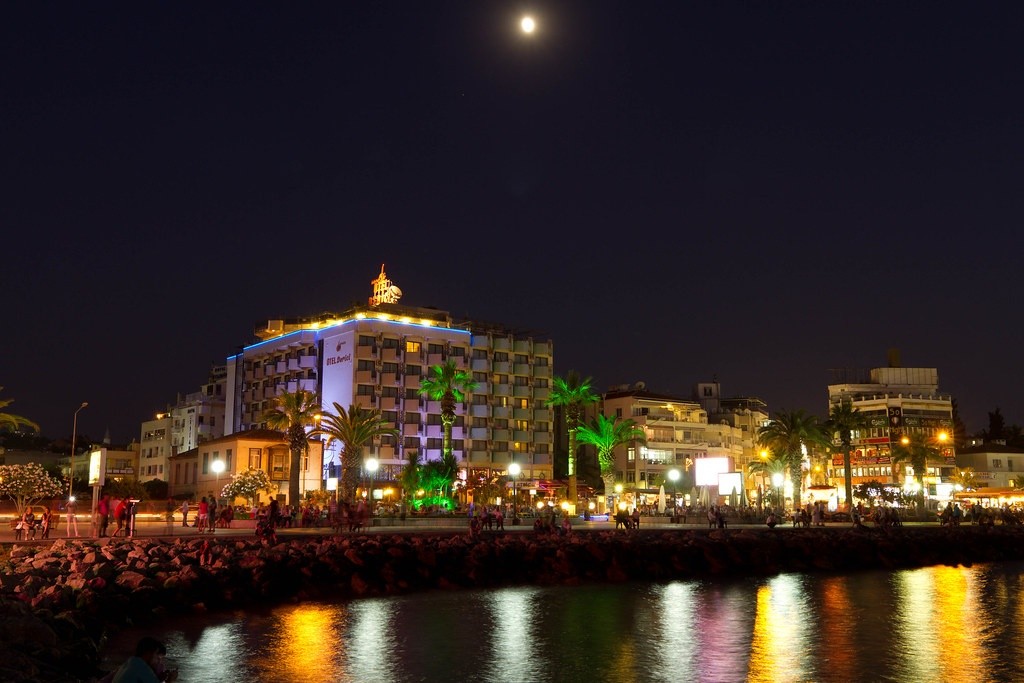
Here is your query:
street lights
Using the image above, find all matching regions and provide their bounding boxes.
[669,469,679,516]
[616,484,624,512]
[366,458,379,512]
[774,472,784,515]
[68,402,89,502]
[211,460,226,505]
[508,463,521,524]
[636,416,665,489]
[761,449,769,512]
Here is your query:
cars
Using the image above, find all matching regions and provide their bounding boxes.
[58,501,78,512]
[831,512,858,522]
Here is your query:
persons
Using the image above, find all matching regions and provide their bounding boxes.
[253,496,535,532]
[617,508,639,529]
[793,500,826,527]
[664,503,783,517]
[968,500,1024,526]
[767,513,777,527]
[99,494,134,537]
[199,496,235,534]
[182,498,191,527]
[15,515,25,540]
[707,507,721,528]
[637,504,657,517]
[165,498,175,523]
[41,507,53,539]
[939,502,962,527]
[64,496,82,537]
[714,505,728,528]
[22,506,37,541]
[850,501,901,526]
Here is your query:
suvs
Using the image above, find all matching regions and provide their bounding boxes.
[22,506,49,522]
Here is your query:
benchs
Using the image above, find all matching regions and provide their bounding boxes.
[939,516,960,526]
[480,514,507,531]
[708,514,728,529]
[8,519,54,540]
[613,515,640,530]
[873,517,903,527]
[335,518,369,533]
[792,515,813,527]
